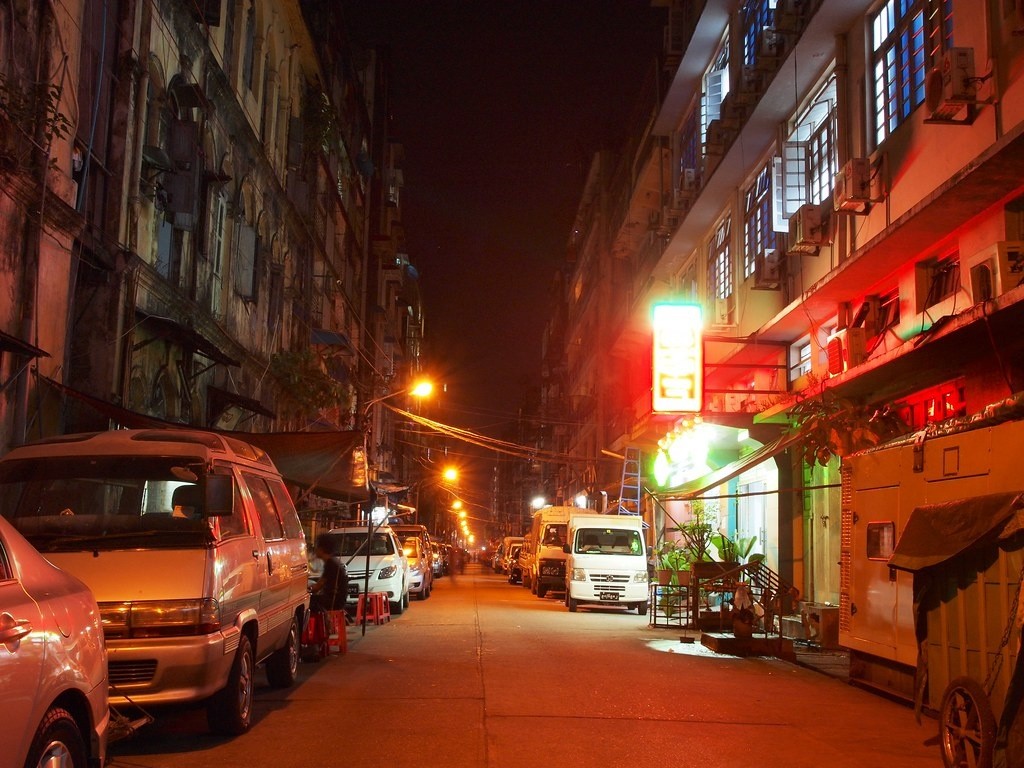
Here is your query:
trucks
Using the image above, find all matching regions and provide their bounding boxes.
[564,513,649,616]
[491,537,522,582]
[525,507,602,599]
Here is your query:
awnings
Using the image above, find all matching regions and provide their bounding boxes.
[207,385,278,423]
[136,309,240,380]
[312,328,355,359]
[0,331,52,391]
[331,364,353,394]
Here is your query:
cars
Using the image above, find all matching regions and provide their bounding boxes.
[430,541,469,579]
[0,514,109,768]
[399,536,432,600]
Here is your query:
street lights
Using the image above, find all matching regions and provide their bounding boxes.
[414,469,458,524]
[429,498,474,549]
[362,380,432,523]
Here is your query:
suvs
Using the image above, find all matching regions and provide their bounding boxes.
[306,526,410,615]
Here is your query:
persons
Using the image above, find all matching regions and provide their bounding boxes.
[308,534,349,615]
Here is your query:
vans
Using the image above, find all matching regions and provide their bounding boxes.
[389,525,434,576]
[0,427,308,739]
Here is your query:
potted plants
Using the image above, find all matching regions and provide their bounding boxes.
[673,548,694,586]
[672,496,741,579]
[652,539,674,585]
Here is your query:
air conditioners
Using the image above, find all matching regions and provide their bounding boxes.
[705,298,728,324]
[961,241,1024,312]
[827,328,866,379]
[925,47,977,120]
[833,158,870,212]
[659,1,799,237]
[754,248,778,288]
[788,204,821,253]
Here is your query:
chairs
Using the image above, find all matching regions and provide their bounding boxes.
[582,535,601,548]
[612,536,631,552]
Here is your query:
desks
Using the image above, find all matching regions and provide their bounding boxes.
[649,584,696,629]
[693,585,751,634]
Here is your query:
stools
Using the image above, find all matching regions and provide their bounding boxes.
[368,592,390,624]
[356,593,385,626]
[318,609,347,657]
[301,610,321,645]
